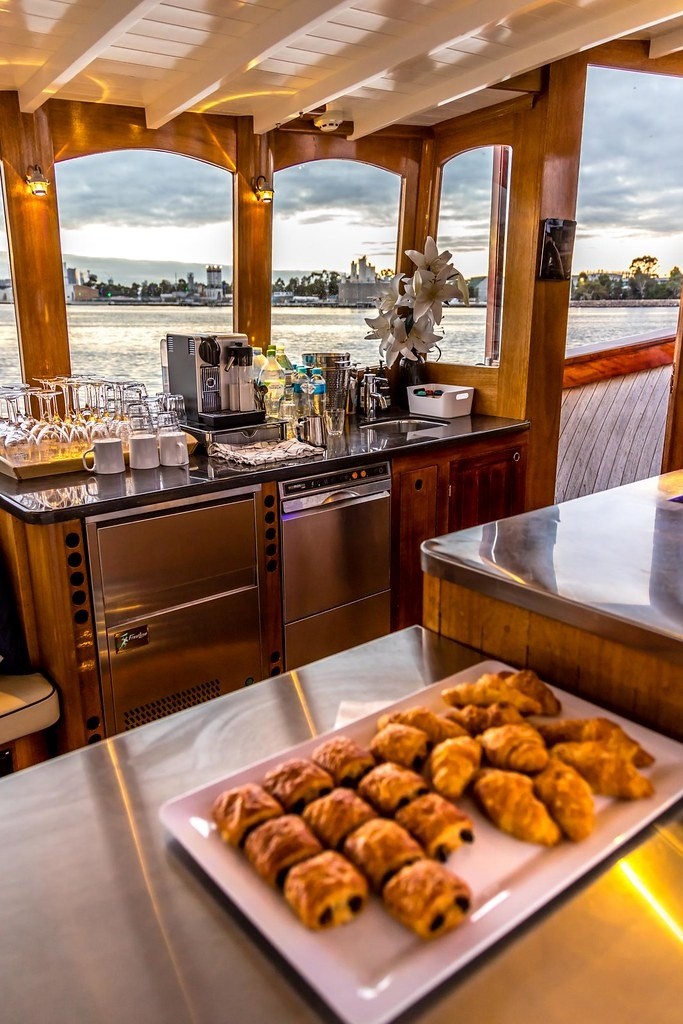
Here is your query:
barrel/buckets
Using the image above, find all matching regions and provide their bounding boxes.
[302,353,351,413]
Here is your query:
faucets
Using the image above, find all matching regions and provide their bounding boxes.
[364,374,388,421]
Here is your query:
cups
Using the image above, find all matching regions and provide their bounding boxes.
[82,438,126,474]
[159,432,190,466]
[128,434,160,469]
[295,415,324,446]
[323,408,344,437]
[101,375,186,434]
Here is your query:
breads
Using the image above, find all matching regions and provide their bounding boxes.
[212,669,658,939]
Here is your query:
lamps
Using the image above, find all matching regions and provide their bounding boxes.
[254,175,275,202]
[27,165,51,197]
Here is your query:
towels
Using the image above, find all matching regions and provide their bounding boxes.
[207,438,325,474]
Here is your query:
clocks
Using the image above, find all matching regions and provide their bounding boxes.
[539,219,578,280]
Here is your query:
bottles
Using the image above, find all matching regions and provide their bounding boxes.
[253,344,326,440]
[417,389,444,398]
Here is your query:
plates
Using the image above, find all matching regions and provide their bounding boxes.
[158,660,683,1024]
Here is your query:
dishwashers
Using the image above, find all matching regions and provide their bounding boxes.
[278,460,392,672]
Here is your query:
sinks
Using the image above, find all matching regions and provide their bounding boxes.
[358,416,450,434]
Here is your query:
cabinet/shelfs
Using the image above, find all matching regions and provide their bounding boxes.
[391,431,528,632]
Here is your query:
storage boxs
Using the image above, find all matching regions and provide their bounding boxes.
[407,383,473,418]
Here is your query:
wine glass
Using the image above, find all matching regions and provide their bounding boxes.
[0,372,134,467]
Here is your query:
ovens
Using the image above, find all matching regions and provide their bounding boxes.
[84,483,271,738]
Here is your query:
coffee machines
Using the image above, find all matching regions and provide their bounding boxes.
[160,332,266,428]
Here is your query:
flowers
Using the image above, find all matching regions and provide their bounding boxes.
[365,236,486,370]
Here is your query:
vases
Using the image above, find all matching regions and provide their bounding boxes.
[399,350,425,408]
